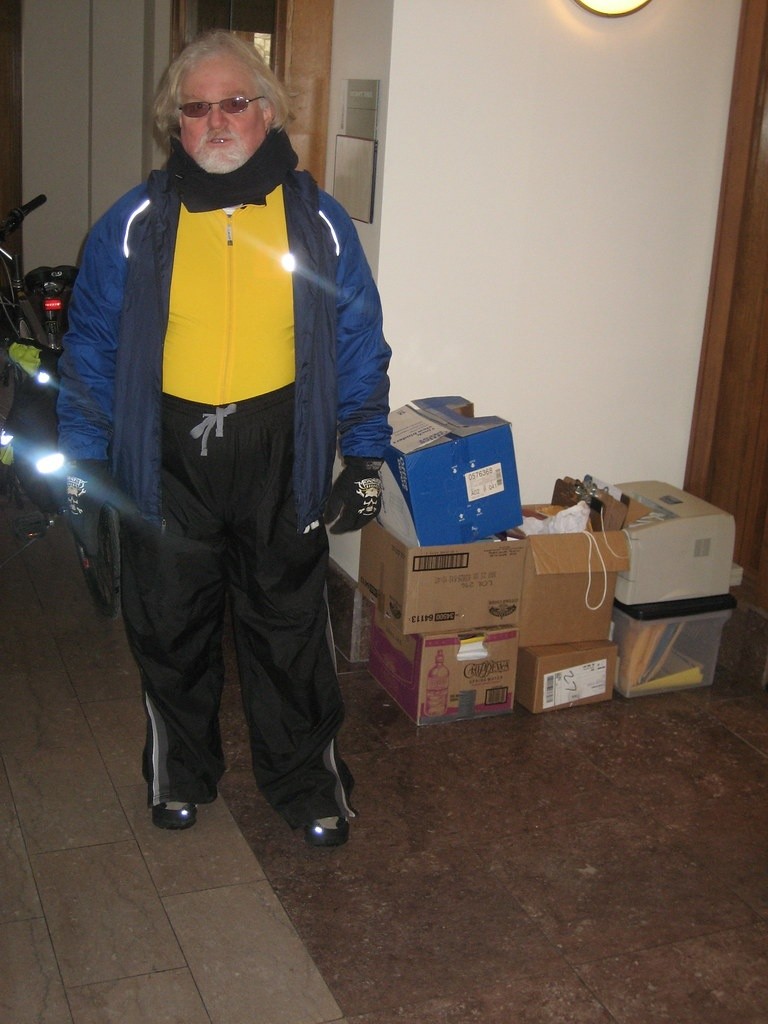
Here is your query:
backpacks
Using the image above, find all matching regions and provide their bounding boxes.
[0,339,60,515]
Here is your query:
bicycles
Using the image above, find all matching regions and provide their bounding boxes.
[0,193,122,627]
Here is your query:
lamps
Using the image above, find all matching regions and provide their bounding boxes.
[577,0,651,17]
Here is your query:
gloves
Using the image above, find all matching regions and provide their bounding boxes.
[65,458,141,556]
[321,455,385,534]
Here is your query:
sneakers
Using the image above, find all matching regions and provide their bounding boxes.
[307,811,350,846]
[152,795,196,830]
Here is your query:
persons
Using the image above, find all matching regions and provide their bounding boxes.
[55,28,391,847]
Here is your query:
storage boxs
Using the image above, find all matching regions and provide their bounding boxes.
[359,397,745,730]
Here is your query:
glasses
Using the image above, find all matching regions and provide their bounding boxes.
[179,95,267,119]
